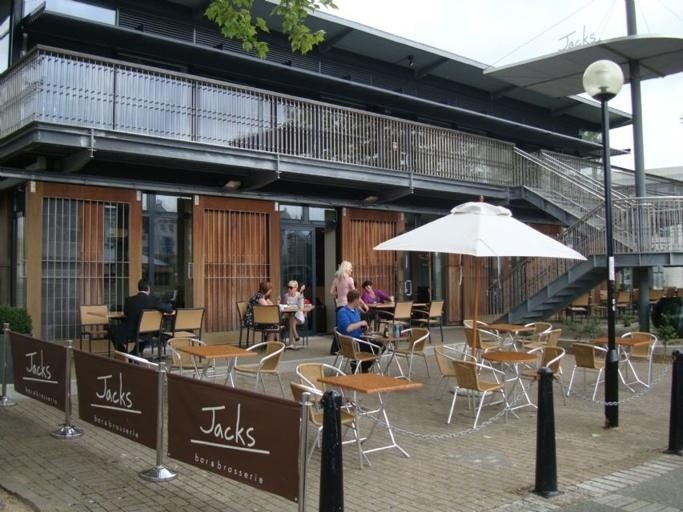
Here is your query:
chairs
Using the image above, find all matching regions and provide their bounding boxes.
[235,300,315,352]
[289,381,364,470]
[333,326,431,381]
[78,302,207,361]
[518,345,568,407]
[295,361,356,447]
[334,297,446,344]
[564,287,683,323]
[113,350,159,369]
[567,342,608,401]
[234,341,286,396]
[446,360,508,429]
[433,344,477,400]
[617,332,658,389]
[167,338,216,377]
[462,318,553,362]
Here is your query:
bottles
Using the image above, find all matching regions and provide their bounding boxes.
[384,324,400,338]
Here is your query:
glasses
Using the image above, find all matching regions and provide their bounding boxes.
[287,286,293,290]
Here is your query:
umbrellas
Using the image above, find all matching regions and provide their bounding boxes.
[372,193,589,361]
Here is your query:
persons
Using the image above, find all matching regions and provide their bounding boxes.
[337,288,386,374]
[284,279,306,348]
[111,278,174,363]
[330,260,356,354]
[361,280,393,321]
[243,281,281,341]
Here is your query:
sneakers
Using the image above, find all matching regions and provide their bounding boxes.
[349,360,356,374]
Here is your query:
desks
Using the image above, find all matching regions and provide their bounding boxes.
[317,372,423,467]
[592,337,651,393]
[481,352,537,419]
[175,345,257,388]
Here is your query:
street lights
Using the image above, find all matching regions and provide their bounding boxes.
[582,60,624,426]
